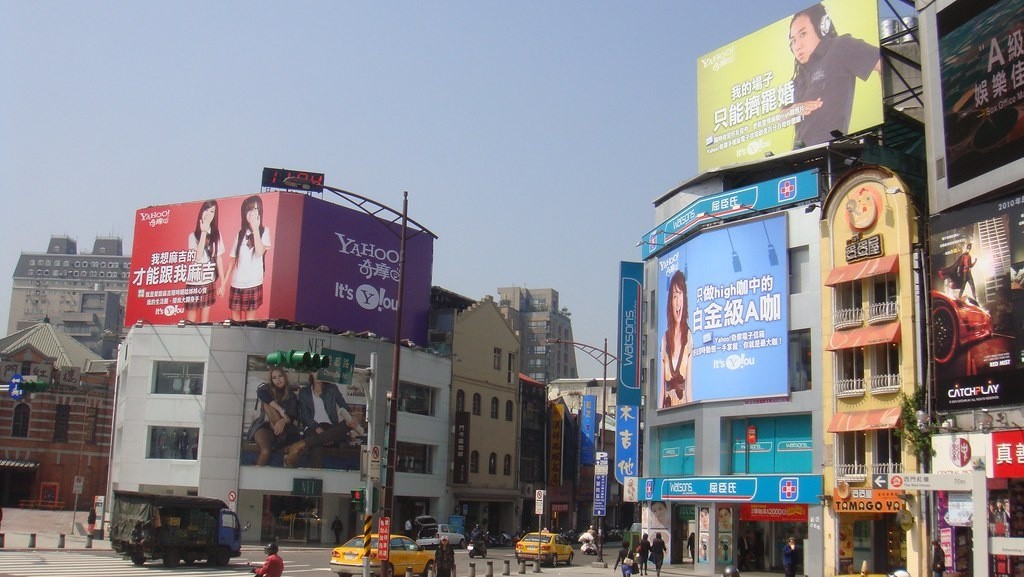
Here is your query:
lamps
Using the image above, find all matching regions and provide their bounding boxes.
[222,316,243,329]
[897,493,913,503]
[974,412,1024,434]
[816,495,832,508]
[763,127,869,179]
[635,204,767,248]
[313,325,330,332]
[805,204,822,214]
[885,187,926,201]
[355,330,462,361]
[915,410,968,434]
[135,318,153,328]
[338,330,355,336]
[265,321,276,329]
[177,318,201,329]
[99,328,126,345]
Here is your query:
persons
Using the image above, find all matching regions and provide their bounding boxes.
[658,270,696,410]
[87,506,95,537]
[648,532,667,577]
[931,541,944,577]
[248,365,299,467]
[718,508,731,529]
[637,534,650,576]
[700,507,709,530]
[185,199,225,324]
[649,501,670,527]
[988,499,1011,537]
[217,195,271,320]
[471,523,485,553]
[404,518,413,536]
[432,535,455,577]
[776,3,879,151]
[613,540,634,577]
[738,531,750,571]
[157,429,197,460]
[686,533,694,564]
[251,543,283,577]
[331,516,344,545]
[285,370,358,468]
[783,537,798,577]
[586,525,597,536]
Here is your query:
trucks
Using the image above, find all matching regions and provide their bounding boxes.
[109,490,252,567]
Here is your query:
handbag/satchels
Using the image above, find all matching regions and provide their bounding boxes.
[624,557,633,566]
[636,546,642,553]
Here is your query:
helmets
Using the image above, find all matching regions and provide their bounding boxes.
[265,543,278,555]
[590,525,594,529]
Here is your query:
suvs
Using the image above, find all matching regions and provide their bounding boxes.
[415,515,466,549]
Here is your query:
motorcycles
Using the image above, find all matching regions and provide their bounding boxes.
[467,532,489,558]
[483,529,513,548]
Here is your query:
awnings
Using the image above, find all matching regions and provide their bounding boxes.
[827,407,905,433]
[824,322,900,352]
[824,254,899,287]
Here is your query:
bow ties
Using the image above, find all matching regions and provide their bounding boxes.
[205,234,211,246]
[246,233,254,249]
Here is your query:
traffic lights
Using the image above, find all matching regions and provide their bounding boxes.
[350,490,365,514]
[291,351,330,370]
[18,381,48,393]
[266,353,278,365]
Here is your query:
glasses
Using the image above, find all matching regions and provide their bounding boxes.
[247,208,257,214]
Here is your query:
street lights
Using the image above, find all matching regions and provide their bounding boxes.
[282,176,407,577]
[543,336,608,561]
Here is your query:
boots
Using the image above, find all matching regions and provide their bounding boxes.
[284,439,309,467]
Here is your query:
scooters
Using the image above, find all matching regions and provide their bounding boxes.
[585,536,599,555]
[513,525,627,544]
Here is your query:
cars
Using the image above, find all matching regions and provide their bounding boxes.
[515,531,575,568]
[330,532,436,577]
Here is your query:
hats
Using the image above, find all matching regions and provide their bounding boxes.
[441,535,448,541]
